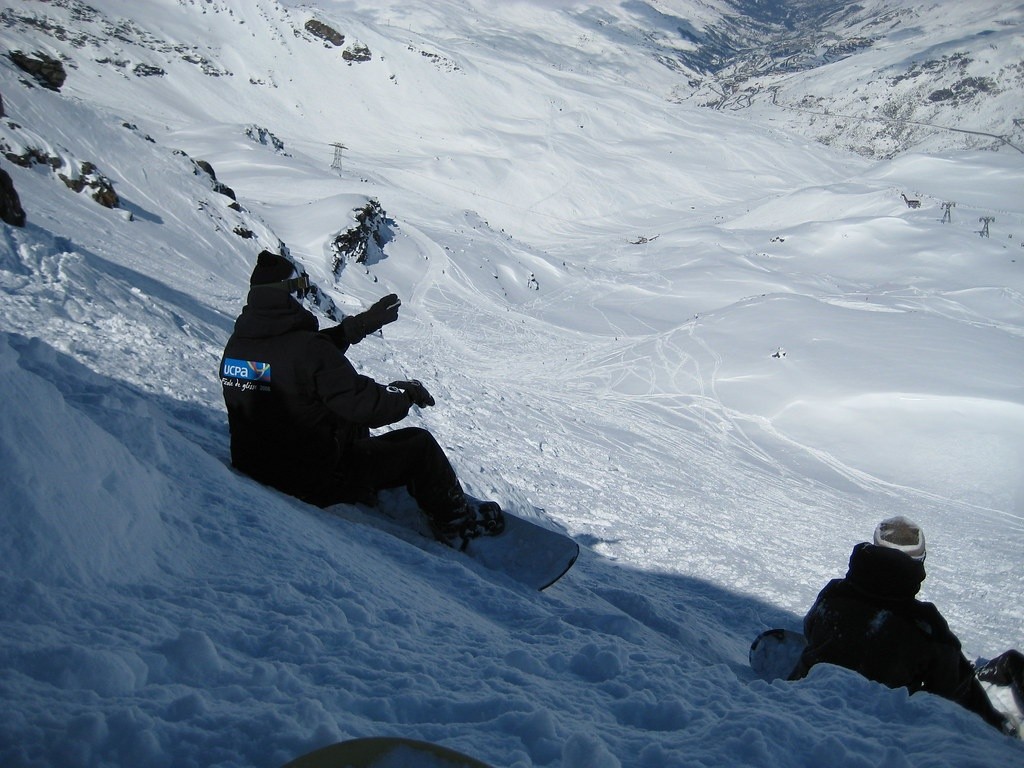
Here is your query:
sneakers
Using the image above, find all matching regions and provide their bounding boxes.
[431,501,504,545]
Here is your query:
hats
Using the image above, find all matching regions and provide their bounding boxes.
[250,250,295,287]
[874,516,927,563]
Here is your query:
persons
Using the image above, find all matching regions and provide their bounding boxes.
[217,249,502,538]
[784,513,1024,736]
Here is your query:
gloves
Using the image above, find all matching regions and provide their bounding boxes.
[982,704,1008,731]
[344,294,401,345]
[389,379,435,408]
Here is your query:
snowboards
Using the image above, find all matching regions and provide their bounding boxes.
[749,628,806,680]
[355,483,579,591]
